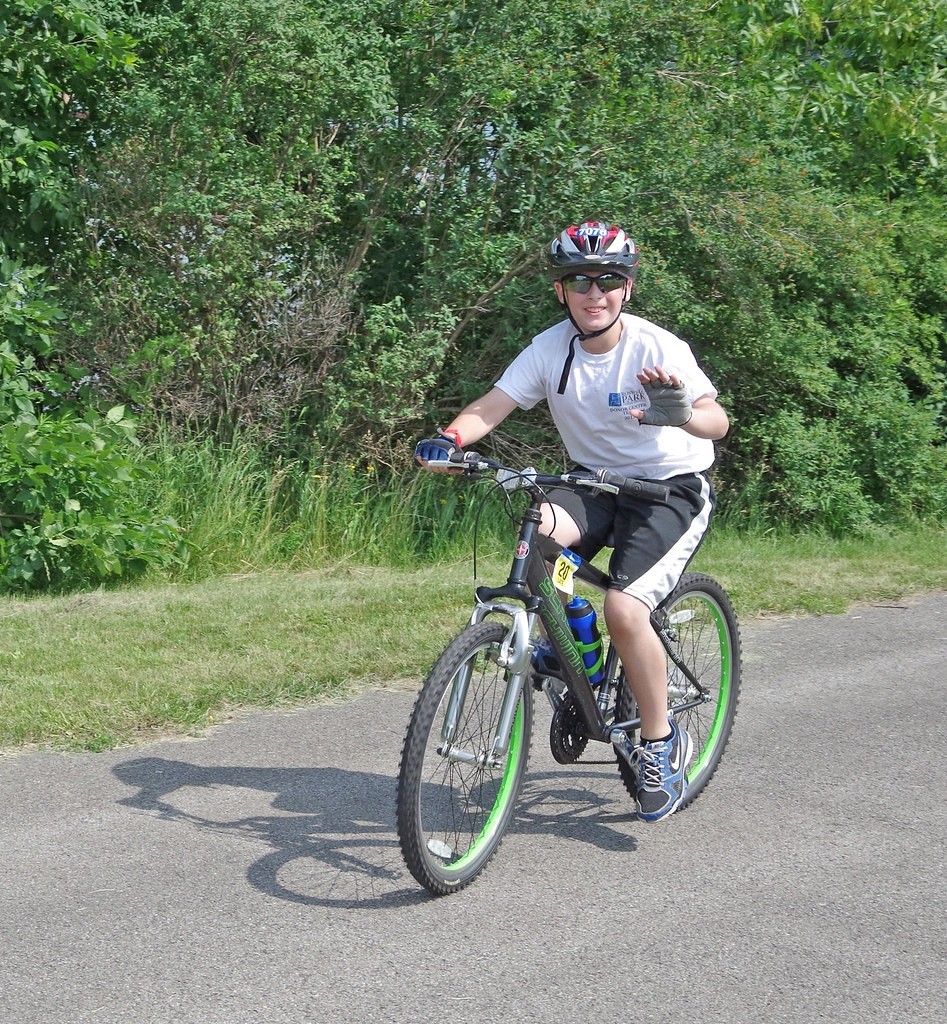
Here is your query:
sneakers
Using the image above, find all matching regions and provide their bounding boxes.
[491,636,567,695]
[628,718,693,823]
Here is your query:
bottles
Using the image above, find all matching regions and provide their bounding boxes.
[564,596,605,683]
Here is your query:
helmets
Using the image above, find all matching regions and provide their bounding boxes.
[547,221,639,281]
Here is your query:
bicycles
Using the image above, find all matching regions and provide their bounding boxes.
[392,452,743,893]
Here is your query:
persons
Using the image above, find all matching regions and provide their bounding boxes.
[411,224,731,822]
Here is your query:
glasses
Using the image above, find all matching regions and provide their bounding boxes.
[557,273,627,294]
